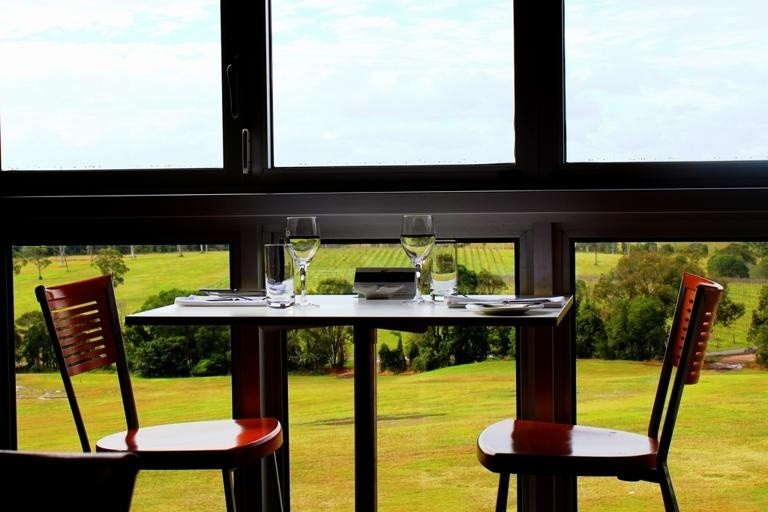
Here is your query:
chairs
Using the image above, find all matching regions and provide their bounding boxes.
[36,275,285,511]
[476,272,725,510]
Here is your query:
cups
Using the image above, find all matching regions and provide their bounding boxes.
[264,244,295,308]
[429,240,457,302]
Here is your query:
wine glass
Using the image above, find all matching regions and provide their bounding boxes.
[284,216,321,309]
[400,214,436,305]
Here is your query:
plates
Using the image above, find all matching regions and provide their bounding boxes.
[465,303,531,315]
